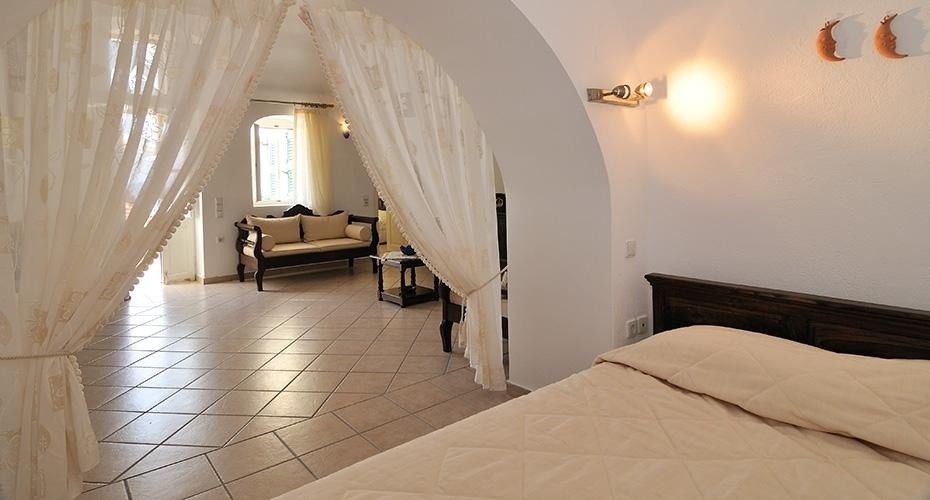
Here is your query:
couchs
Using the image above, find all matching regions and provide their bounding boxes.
[234,204,381,291]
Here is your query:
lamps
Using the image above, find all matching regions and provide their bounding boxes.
[588,80,653,108]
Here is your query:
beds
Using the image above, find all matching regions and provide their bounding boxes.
[268,268,929,500]
